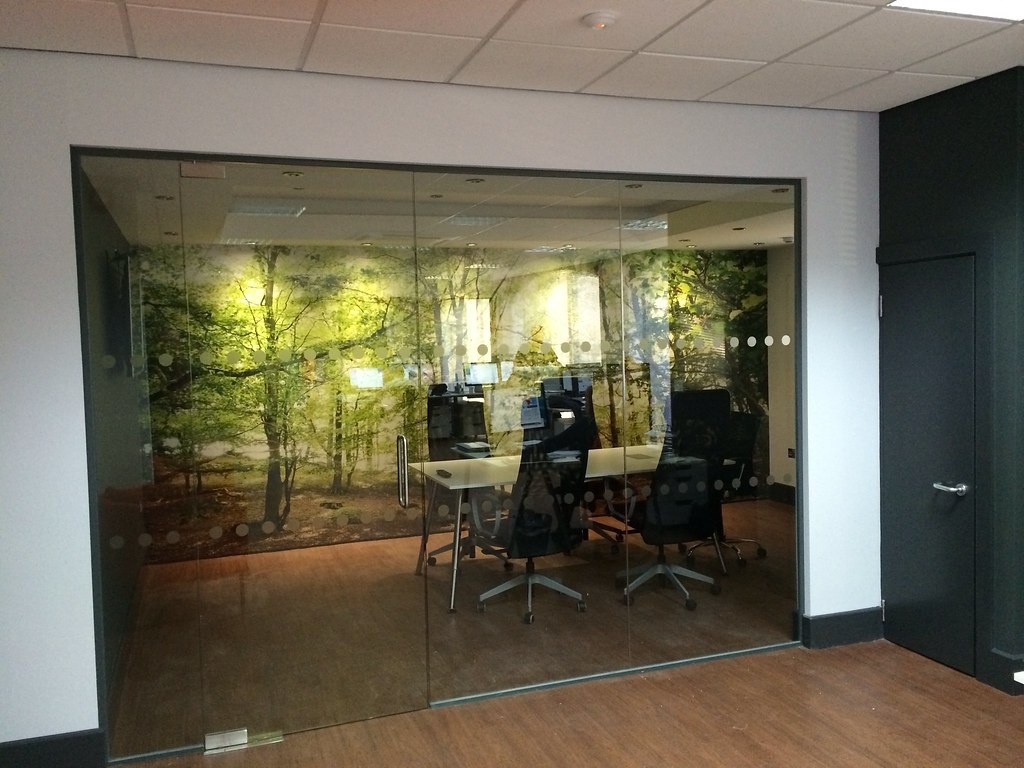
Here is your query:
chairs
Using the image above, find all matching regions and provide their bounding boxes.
[603,389,731,610]
[475,395,595,625]
[426,382,514,572]
[541,377,624,554]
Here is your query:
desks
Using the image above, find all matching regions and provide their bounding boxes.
[407,443,736,613]
